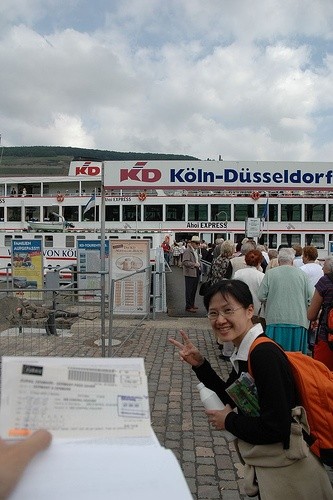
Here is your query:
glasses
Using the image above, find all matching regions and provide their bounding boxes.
[205,306,244,318]
[194,242,201,245]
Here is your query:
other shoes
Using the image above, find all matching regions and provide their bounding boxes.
[219,353,232,362]
[193,306,198,309]
[217,341,224,349]
[186,309,197,313]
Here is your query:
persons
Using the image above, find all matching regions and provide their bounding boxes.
[299,246,324,350]
[322,256,333,275]
[308,275,333,372]
[182,236,203,313]
[161,241,186,270]
[219,249,265,362]
[169,280,333,500]
[256,249,314,355]
[201,239,321,350]
[0,429,52,500]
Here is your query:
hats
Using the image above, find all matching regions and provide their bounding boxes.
[187,236,202,242]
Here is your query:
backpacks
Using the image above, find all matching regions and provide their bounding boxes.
[324,272,333,351]
[247,335,333,468]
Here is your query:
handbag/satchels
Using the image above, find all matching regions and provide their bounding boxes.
[226,371,264,422]
[199,280,209,296]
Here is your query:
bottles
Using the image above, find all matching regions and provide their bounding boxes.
[196,382,237,442]
